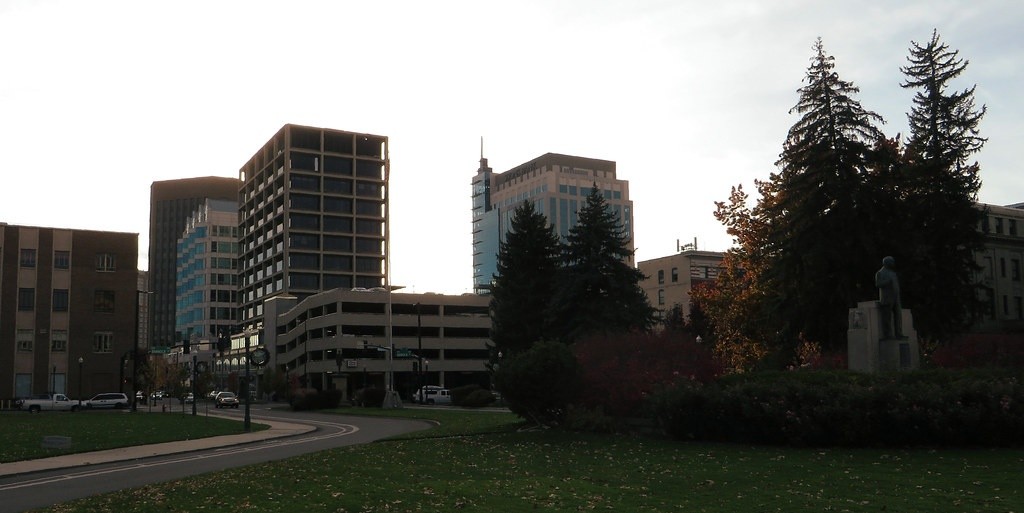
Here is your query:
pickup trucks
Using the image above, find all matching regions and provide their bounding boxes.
[20,394,79,413]
[415,386,456,405]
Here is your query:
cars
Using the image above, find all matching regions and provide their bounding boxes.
[214,392,240,408]
[181,392,195,404]
[150,391,163,400]
[134,392,146,401]
[210,391,219,400]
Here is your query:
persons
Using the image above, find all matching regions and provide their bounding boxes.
[335,349,345,374]
[875,256,909,342]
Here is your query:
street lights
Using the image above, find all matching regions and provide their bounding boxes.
[191,348,198,416]
[78,354,84,407]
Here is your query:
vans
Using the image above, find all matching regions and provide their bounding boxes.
[80,392,129,410]
